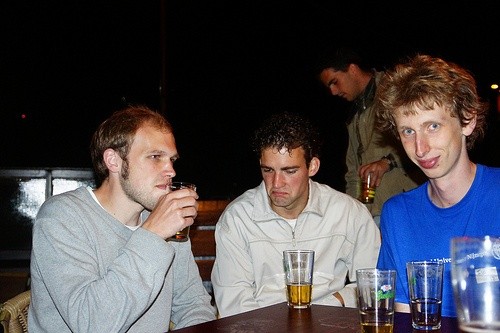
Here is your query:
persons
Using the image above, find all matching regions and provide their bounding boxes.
[372,54,500,321]
[27,107,218,333]
[316,42,424,230]
[212,113,381,318]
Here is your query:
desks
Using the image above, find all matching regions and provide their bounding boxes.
[167,302,467,333]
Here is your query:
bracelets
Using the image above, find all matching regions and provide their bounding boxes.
[389,153,398,168]
[383,155,394,171]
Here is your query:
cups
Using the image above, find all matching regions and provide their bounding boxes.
[356,268,396,333]
[449,237,500,333]
[166,182,196,243]
[406,261,445,331]
[361,172,377,203]
[283,251,315,308]
